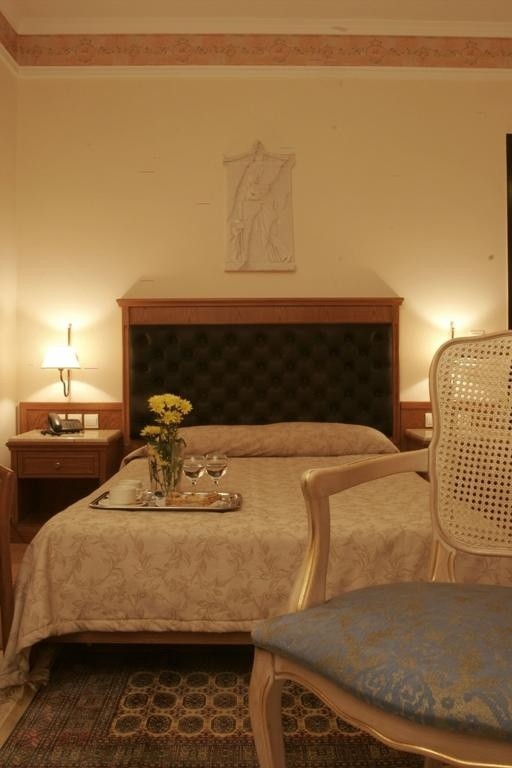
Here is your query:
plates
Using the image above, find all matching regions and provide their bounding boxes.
[88,491,242,513]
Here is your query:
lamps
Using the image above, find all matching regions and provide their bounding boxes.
[40,323,82,397]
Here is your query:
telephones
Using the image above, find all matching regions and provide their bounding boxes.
[48,412,83,434]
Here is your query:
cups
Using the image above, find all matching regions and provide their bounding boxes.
[109,480,143,505]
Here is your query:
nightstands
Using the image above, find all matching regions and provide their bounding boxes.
[405,426,432,481]
[6,429,122,543]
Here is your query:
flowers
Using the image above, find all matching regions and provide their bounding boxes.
[140,393,194,500]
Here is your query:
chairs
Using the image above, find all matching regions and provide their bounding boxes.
[247,331,512,767]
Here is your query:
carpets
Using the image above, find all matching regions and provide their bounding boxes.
[1,647,422,767]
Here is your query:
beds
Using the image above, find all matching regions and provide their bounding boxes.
[9,299,477,688]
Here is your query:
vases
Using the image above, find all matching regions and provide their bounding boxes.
[147,446,184,502]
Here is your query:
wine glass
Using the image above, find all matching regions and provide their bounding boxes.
[181,454,205,499]
[205,454,230,494]
